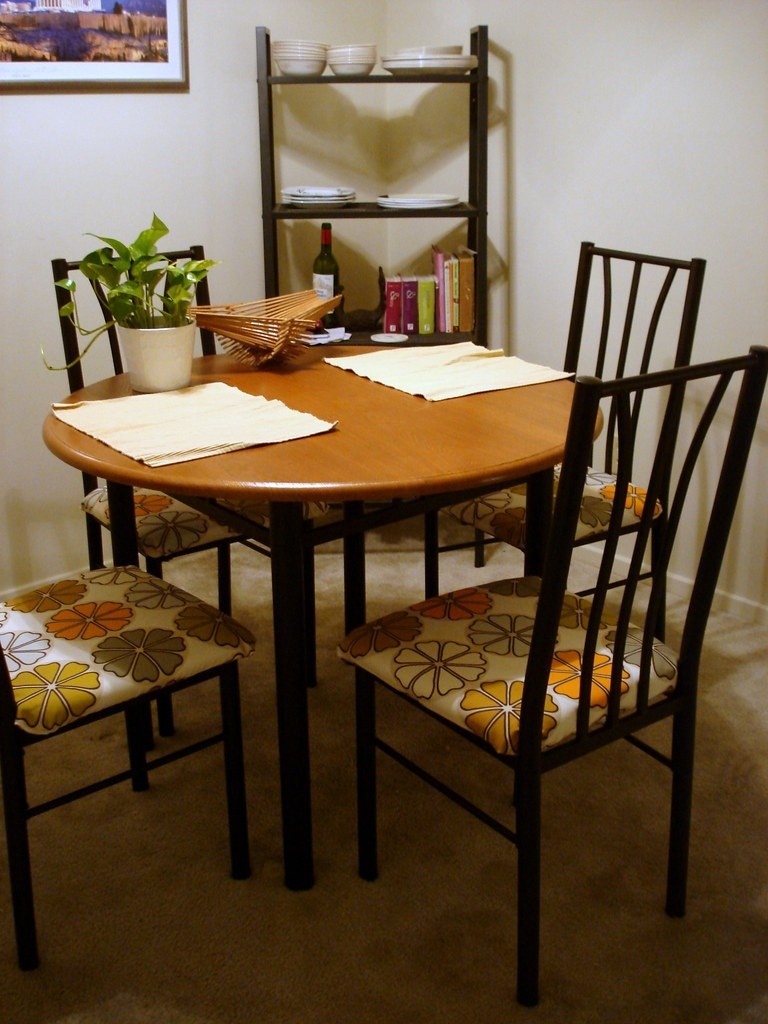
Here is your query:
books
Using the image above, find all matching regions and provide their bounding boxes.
[386,245,474,335]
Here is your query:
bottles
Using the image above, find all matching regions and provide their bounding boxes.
[312,222,339,329]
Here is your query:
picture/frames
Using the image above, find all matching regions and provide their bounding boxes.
[0,0,188,97]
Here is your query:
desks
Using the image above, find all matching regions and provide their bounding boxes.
[43,340,605,894]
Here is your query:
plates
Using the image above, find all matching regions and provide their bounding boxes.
[279,185,357,209]
[380,45,477,74]
[377,194,461,209]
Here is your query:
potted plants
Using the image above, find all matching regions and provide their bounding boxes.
[37,212,216,397]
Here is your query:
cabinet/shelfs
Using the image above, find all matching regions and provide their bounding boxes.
[253,25,489,568]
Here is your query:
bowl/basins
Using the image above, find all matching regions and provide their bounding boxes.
[271,40,377,76]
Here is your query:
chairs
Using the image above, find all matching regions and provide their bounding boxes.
[338,341,767,1007]
[51,245,255,776]
[419,245,710,646]
[1,570,255,969]
